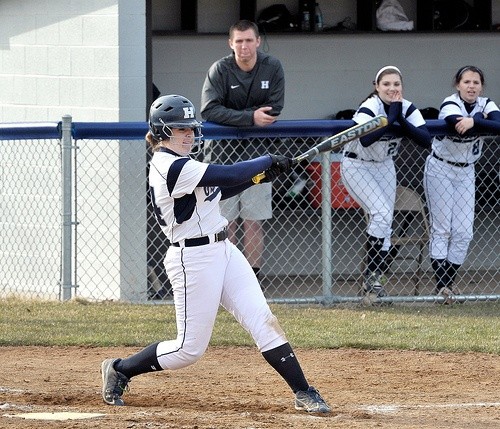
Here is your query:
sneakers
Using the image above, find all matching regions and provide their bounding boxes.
[357,272,384,298]
[102,358,131,406]
[294,386,329,413]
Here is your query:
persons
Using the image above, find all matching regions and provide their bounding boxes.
[100,96,330,414]
[201,21,285,285]
[339,64,427,305]
[422,64,500,302]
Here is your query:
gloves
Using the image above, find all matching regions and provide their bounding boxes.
[258,167,285,183]
[268,153,293,171]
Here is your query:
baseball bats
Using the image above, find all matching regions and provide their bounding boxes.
[250,115,389,184]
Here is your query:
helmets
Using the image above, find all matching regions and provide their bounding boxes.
[149,94,198,127]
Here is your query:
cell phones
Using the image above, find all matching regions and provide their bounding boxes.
[271,113,281,116]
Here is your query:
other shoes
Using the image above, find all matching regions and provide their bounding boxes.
[431,287,456,304]
[450,285,466,303]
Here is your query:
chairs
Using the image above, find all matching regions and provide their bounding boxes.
[357,187,430,296]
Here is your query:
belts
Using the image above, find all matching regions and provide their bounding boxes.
[171,228,228,247]
[432,152,472,167]
[345,151,377,163]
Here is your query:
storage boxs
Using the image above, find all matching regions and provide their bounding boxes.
[308,154,360,208]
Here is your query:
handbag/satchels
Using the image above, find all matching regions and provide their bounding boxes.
[376,0,415,31]
[258,4,299,34]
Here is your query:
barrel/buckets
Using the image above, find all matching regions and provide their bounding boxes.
[306,152,360,209]
